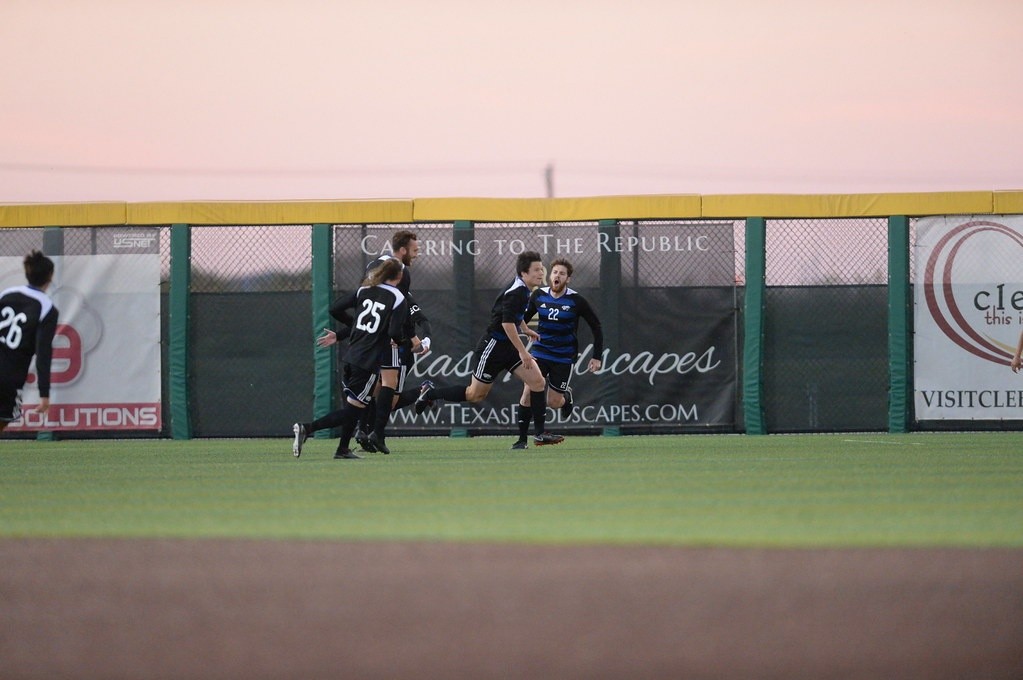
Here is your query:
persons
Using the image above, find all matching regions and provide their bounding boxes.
[511,259,603,449]
[293,231,434,460]
[0,249,59,432]
[414,251,564,446]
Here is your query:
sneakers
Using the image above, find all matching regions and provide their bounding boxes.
[354,430,378,453]
[333,448,359,459]
[366,431,390,455]
[508,441,528,450]
[293,423,307,458]
[420,380,434,408]
[415,383,434,415]
[561,387,573,419]
[534,431,564,446]
